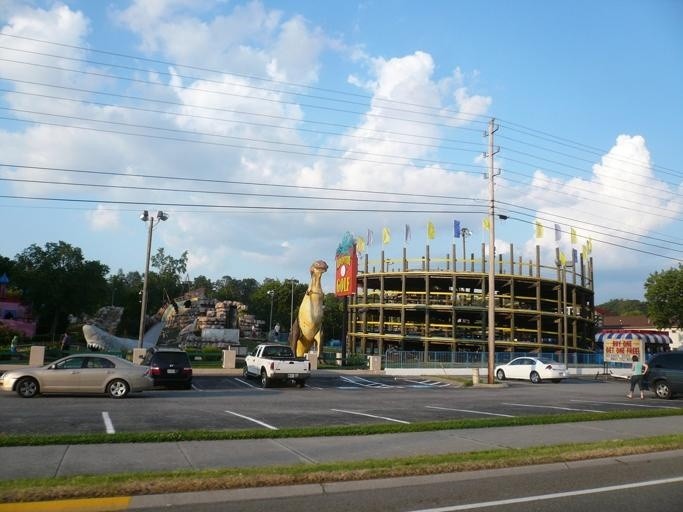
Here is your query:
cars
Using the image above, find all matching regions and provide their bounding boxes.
[0,346,193,399]
[642,352,683,399]
[494,356,568,384]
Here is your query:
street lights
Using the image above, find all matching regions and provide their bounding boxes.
[460,227,473,272]
[138,210,170,348]
[266,290,274,341]
[285,278,299,346]
[554,258,574,370]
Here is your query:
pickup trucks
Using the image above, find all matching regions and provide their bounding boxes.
[243,344,311,388]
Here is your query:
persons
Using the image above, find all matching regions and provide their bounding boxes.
[274,323,280,336]
[251,323,257,338]
[624,354,648,400]
[9,334,18,352]
[60,332,70,350]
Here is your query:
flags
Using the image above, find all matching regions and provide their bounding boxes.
[353,218,594,268]
